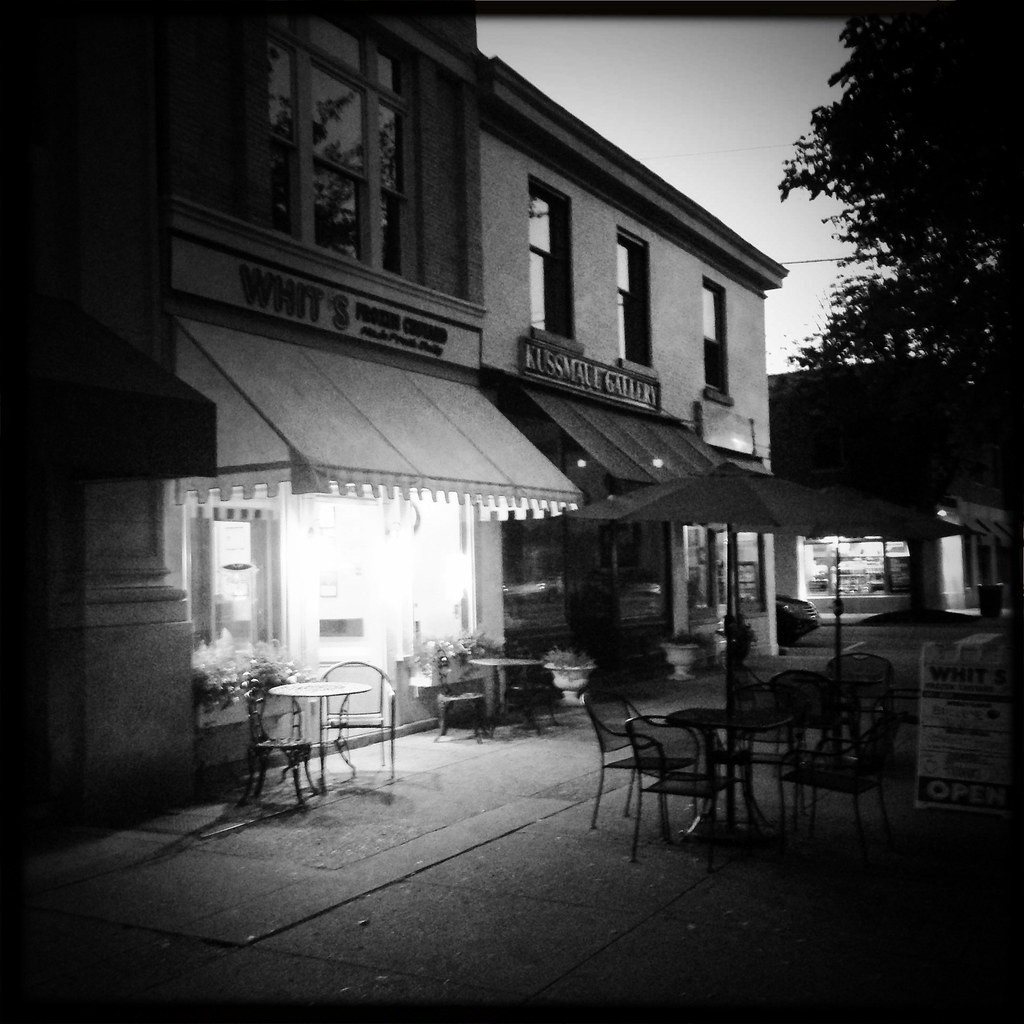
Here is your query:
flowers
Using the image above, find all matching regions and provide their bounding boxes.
[413,632,506,691]
[191,639,300,713]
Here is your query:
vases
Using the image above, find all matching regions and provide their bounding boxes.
[195,688,307,730]
[408,654,493,688]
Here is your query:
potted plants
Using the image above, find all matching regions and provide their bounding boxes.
[658,629,716,681]
[542,646,597,706]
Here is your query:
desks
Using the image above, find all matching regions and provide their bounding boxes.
[665,707,794,853]
[467,658,544,740]
[268,681,372,797]
[830,678,893,764]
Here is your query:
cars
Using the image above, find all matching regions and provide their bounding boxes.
[775,595,822,643]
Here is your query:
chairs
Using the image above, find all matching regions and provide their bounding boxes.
[236,685,320,810]
[318,660,397,780]
[584,651,919,874]
[499,647,564,727]
[434,648,492,745]
